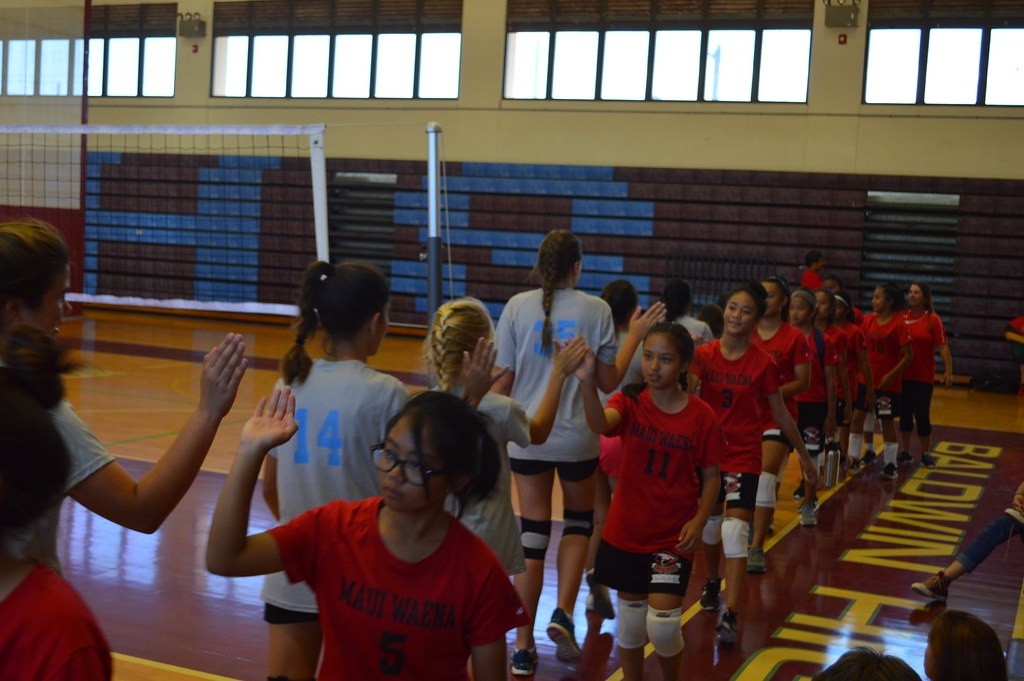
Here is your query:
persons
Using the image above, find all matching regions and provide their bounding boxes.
[265,261,413,681]
[559,251,952,681]
[489,230,668,675]
[910,470,1024,602]
[422,296,588,577]
[0,218,248,681]
[811,610,1022,681]
[206,385,527,681]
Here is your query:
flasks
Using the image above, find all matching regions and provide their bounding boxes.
[822,440,841,489]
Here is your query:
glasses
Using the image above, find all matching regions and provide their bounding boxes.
[370,442,446,487]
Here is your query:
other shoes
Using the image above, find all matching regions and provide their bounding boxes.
[839,456,848,474]
[586,568,614,619]
[586,595,595,611]
[849,458,861,474]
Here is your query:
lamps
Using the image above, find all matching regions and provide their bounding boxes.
[175,10,206,38]
[823,0,859,28]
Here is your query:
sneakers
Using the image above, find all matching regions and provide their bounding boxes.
[878,463,899,479]
[896,450,914,464]
[799,504,817,525]
[859,450,878,464]
[546,607,581,662]
[911,571,950,602]
[768,512,774,530]
[793,480,806,499]
[716,607,738,649]
[510,645,539,675]
[700,577,721,610]
[908,600,947,626]
[746,546,768,573]
[920,451,935,468]
